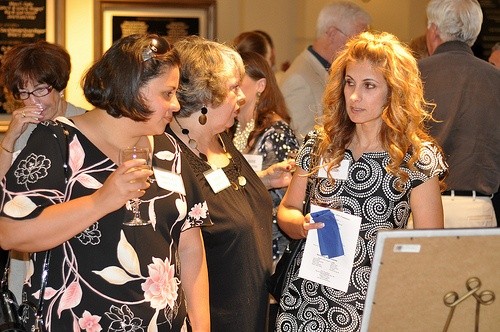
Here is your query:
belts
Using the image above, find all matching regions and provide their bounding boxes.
[441,190,492,197]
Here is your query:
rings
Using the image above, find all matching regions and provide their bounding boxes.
[23,112,26,117]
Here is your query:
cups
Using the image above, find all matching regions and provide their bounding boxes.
[288,151,297,173]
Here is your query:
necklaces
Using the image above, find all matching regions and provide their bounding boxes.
[173,114,247,190]
[232,119,254,151]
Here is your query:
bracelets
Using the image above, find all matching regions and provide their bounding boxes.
[0,144,12,153]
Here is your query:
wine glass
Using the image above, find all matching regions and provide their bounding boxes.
[119,148,152,226]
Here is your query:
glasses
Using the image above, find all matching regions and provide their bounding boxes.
[142,36,171,61]
[13,85,54,100]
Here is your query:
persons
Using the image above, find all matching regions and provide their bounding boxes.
[158,33,272,332]
[276,31,449,332]
[0,31,209,332]
[224,0,500,274]
[0,40,88,181]
